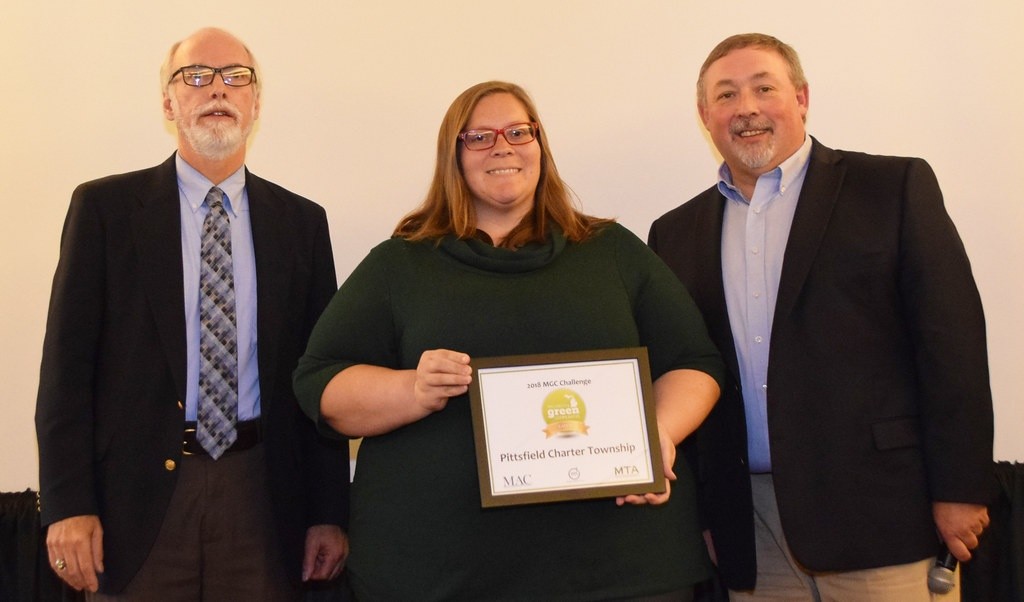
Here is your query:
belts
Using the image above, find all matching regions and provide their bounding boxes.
[181,420,263,456]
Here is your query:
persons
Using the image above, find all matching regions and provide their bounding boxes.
[33,25,352,602]
[643,32,993,601]
[296,81,727,602]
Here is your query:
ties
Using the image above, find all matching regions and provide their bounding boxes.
[195,187,238,461]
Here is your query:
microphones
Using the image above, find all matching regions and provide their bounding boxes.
[928,546,959,595]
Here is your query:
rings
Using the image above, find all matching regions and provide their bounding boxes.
[55,559,66,570]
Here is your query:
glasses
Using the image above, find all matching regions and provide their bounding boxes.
[168,65,257,87]
[458,122,538,151]
